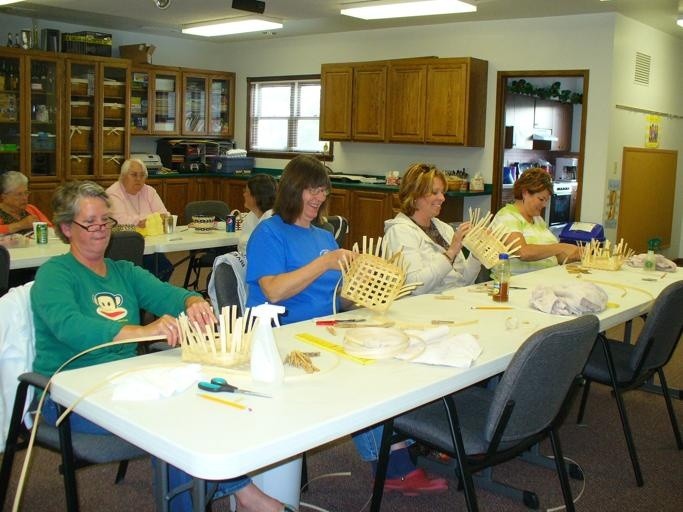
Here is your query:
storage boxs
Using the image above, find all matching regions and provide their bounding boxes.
[119,43,156,64]
[61,31,112,58]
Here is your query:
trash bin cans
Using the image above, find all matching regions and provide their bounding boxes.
[558,222,606,248]
[229,453,303,512]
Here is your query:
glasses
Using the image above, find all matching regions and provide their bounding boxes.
[305,186,333,197]
[70,217,119,233]
[242,187,251,198]
[2,190,34,199]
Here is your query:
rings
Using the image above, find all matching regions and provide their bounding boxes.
[202,311,210,317]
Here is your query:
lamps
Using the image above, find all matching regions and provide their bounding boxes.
[338,0,478,21]
[182,14,283,37]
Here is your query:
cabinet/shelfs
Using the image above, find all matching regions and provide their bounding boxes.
[355,62,387,138]
[0,49,26,176]
[350,192,389,251]
[388,61,426,138]
[211,74,237,134]
[26,52,63,179]
[166,180,194,224]
[197,179,226,202]
[132,67,153,134]
[426,56,489,143]
[503,91,571,150]
[183,73,209,134]
[328,190,350,249]
[103,63,129,174]
[155,69,182,133]
[29,185,62,218]
[226,182,250,214]
[320,63,355,137]
[145,182,163,202]
[66,59,100,176]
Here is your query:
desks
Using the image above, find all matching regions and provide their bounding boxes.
[0,220,241,271]
[48,254,683,512]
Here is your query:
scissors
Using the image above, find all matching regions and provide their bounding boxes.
[316,318,365,326]
[198,377,273,398]
[566,265,592,274]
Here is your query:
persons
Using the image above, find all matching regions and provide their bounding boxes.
[380,164,492,296]
[246,155,449,496]
[105,158,172,226]
[491,169,589,278]
[238,174,276,256]
[0,171,53,236]
[29,181,294,512]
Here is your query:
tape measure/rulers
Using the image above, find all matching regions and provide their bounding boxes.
[294,332,375,364]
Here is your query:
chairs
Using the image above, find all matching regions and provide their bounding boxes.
[0,281,152,511]
[326,215,348,247]
[210,251,248,333]
[0,245,10,296]
[366,314,599,512]
[558,278,682,486]
[184,200,230,290]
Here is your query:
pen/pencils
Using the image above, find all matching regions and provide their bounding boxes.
[470,306,515,310]
[660,273,667,279]
[195,391,252,412]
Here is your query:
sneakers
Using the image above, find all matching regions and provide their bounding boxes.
[367,469,448,495]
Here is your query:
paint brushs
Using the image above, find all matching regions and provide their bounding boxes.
[468,283,528,292]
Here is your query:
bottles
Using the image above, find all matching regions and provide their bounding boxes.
[226,215,236,232]
[0,60,19,89]
[163,216,173,233]
[36,222,48,246]
[14,33,21,46]
[493,254,509,302]
[7,33,12,46]
[644,250,655,272]
